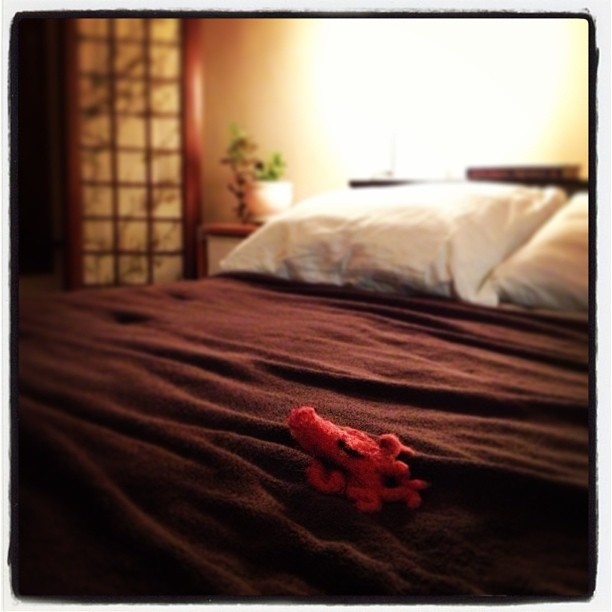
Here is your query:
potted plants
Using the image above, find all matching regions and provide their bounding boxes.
[240,154,293,215]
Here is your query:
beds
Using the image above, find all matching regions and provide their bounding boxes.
[12,174,595,598]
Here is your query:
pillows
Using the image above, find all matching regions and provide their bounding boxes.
[216,178,568,310]
[485,191,590,309]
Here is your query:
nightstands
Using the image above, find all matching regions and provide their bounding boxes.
[196,220,256,279]
